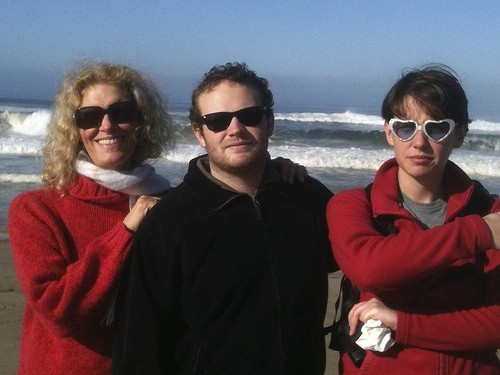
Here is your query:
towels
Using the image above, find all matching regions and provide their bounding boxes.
[355,319,396,352]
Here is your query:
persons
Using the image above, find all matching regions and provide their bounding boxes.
[8,63,307,375]
[110,62,340,375]
[325,63,500,375]
